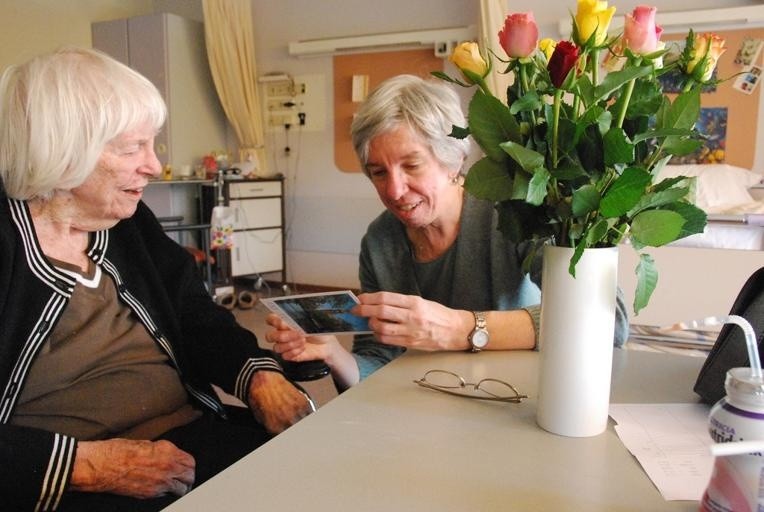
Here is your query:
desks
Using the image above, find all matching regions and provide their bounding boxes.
[158,345,713,512]
[149,176,217,278]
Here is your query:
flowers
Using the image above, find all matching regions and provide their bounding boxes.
[429,0,754,318]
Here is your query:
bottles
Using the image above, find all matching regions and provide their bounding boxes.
[702,364,764,512]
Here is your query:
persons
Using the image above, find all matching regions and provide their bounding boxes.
[263,73,631,395]
[1,42,315,512]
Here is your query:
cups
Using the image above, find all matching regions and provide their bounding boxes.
[194,164,206,179]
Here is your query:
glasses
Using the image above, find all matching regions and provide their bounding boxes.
[414,370,527,404]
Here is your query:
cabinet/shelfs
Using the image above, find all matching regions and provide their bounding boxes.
[258,73,325,133]
[229,180,283,278]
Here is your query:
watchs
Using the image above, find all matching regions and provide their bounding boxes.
[468,309,490,354]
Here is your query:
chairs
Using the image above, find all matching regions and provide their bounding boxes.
[156,215,214,298]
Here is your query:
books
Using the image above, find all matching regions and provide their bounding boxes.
[628,335,714,351]
[628,320,724,346]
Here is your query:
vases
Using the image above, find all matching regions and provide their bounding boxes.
[535,240,620,440]
